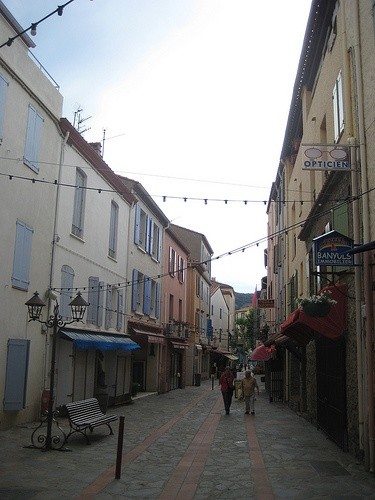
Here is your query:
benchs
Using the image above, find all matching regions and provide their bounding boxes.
[64,398,119,447]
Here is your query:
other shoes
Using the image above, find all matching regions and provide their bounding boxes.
[245,411,249,414]
[251,410,256,415]
[226,409,230,414]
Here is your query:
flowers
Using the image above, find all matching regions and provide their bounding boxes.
[293,290,338,314]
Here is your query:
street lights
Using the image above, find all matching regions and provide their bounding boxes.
[21,289,89,452]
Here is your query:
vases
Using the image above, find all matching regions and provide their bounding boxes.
[303,304,331,317]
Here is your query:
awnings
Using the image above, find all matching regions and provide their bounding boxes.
[206,346,213,351]
[250,345,276,360]
[59,330,140,352]
[223,353,239,360]
[195,345,202,350]
[131,326,164,344]
[279,282,349,347]
[171,340,191,349]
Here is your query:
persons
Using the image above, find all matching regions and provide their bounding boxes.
[219,366,235,415]
[239,362,257,371]
[240,371,259,415]
